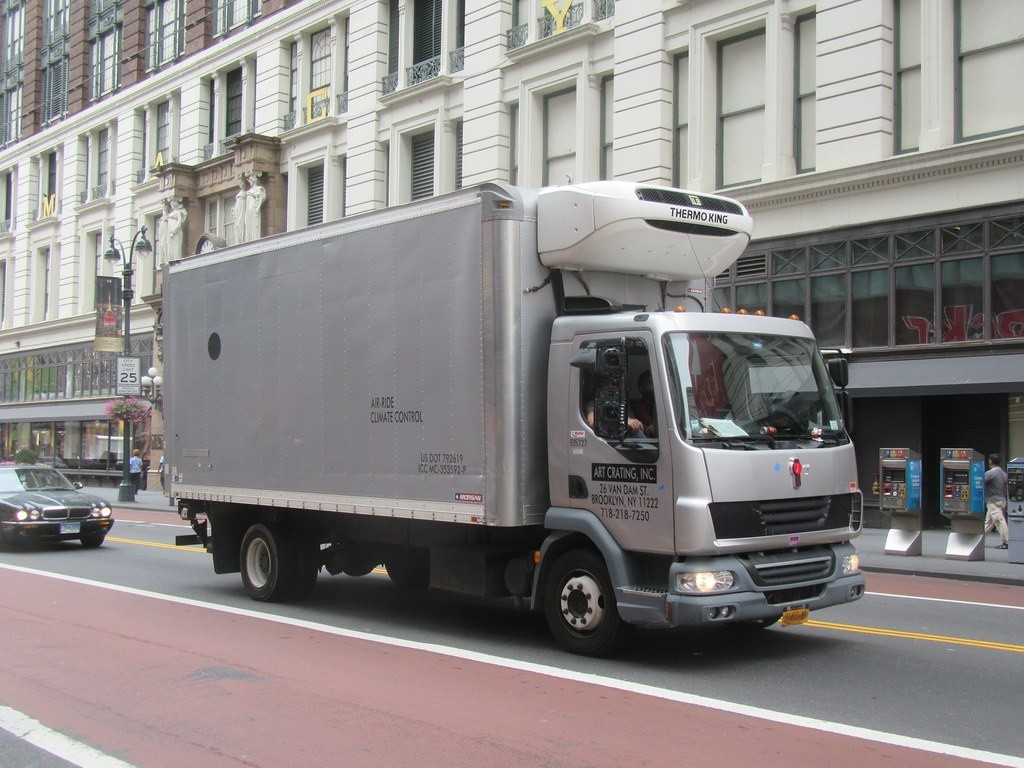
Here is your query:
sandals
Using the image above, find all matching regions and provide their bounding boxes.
[995,543,1007,550]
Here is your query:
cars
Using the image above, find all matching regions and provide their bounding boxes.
[39,456,69,470]
[1,464,115,551]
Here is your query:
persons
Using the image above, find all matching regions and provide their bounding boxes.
[157,455,165,495]
[233,176,267,244]
[130,449,143,495]
[157,201,187,270]
[984,454,1007,549]
[586,370,656,439]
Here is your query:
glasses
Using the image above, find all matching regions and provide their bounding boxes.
[641,378,652,386]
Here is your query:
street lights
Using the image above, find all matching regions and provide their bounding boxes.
[103,226,153,503]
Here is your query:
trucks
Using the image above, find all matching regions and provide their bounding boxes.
[159,180,867,657]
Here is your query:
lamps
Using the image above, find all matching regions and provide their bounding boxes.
[200,238,214,254]
[141,367,163,419]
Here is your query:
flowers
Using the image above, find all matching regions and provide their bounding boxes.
[104,397,152,424]
